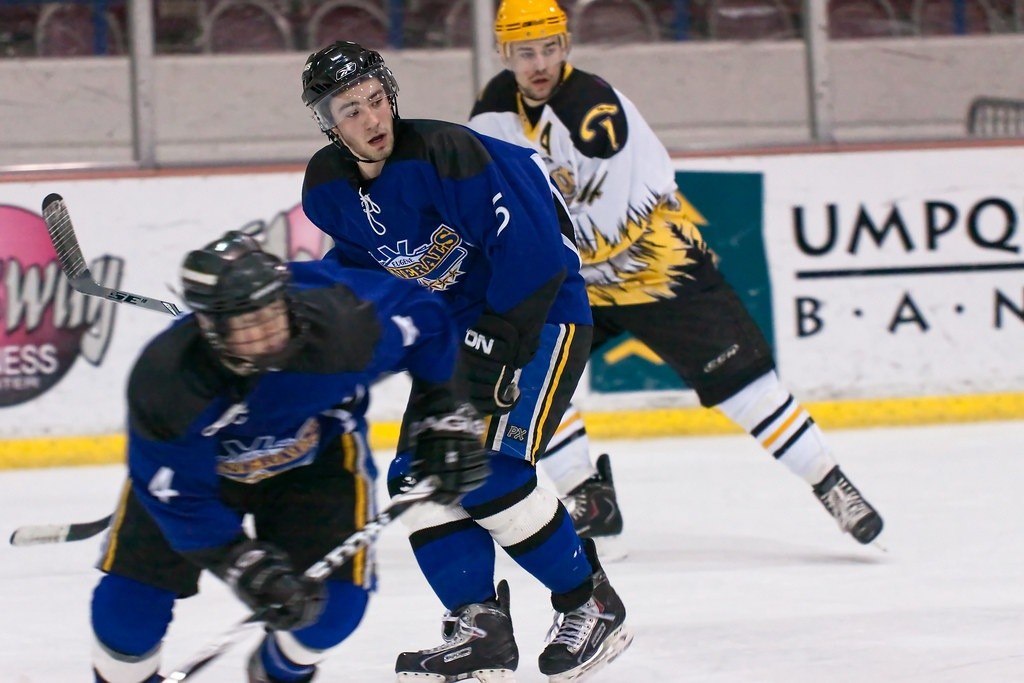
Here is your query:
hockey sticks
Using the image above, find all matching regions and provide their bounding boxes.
[8,513,118,548]
[38,191,190,320]
[165,474,442,683]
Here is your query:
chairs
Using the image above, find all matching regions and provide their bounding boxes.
[0,0,1024,64]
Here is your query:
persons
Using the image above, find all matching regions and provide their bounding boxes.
[466,0,884,546]
[300,37,626,674]
[90,230,488,683]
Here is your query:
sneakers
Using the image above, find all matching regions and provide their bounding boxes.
[539,536,633,683]
[813,462,884,544]
[558,453,623,540]
[395,579,519,683]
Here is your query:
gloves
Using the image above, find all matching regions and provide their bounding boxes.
[403,393,490,507]
[461,312,530,416]
[223,542,325,631]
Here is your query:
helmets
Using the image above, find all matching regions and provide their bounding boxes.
[301,41,392,140]
[493,0,567,50]
[182,230,295,376]
